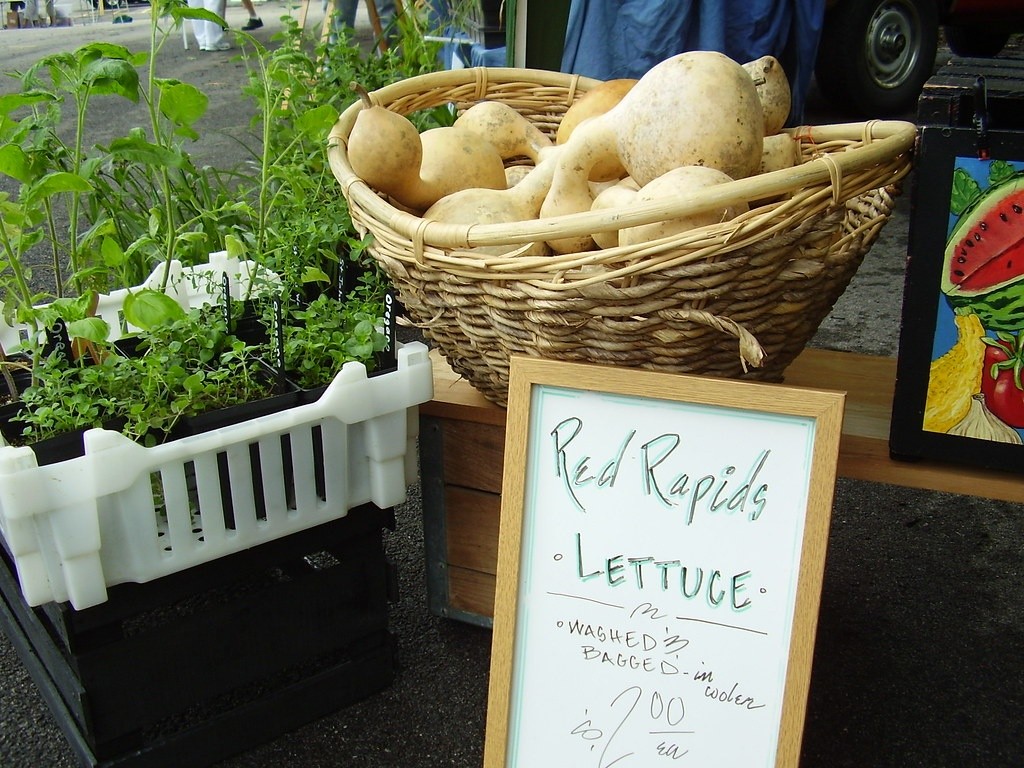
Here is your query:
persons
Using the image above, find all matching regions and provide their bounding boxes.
[188,0,264,52]
[18,1,56,29]
[323,1,397,78]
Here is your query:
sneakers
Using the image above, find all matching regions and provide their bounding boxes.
[199,42,231,53]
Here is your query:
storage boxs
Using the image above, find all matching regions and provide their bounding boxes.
[0,502,398,768]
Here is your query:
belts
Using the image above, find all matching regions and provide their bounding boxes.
[326,65,915,411]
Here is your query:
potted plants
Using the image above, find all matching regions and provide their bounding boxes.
[0,0,496,522]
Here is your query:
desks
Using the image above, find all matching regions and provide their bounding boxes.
[419,344,1024,630]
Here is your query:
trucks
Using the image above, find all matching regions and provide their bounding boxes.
[504,1,1023,120]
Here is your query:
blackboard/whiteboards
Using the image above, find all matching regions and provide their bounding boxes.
[482,353,847,768]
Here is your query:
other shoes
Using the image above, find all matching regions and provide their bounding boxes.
[242,17,264,30]
